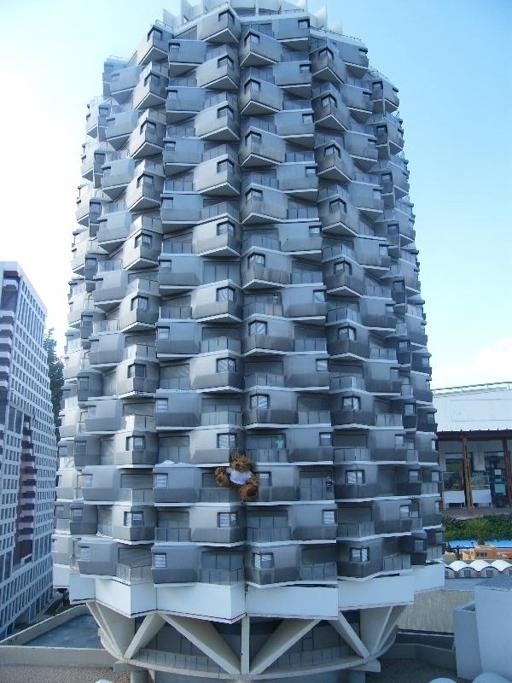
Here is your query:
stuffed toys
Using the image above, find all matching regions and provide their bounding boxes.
[214,453,261,501]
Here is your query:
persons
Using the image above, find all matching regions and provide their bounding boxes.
[476,539,487,556]
[447,471,462,509]
[445,545,456,565]
